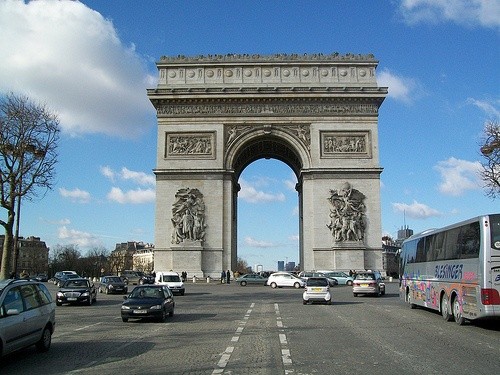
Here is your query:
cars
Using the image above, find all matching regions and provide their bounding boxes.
[56,278,97,306]
[267,272,306,288]
[323,271,353,286]
[303,277,331,305]
[353,270,386,297]
[36,274,48,282]
[54,272,83,288]
[121,285,175,322]
[98,276,128,294]
[236,274,268,286]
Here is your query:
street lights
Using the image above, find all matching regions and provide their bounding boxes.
[1,135,45,279]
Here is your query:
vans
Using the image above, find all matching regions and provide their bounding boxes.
[155,272,185,296]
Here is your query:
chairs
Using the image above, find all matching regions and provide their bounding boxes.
[22,288,33,302]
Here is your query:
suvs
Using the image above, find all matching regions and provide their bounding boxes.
[120,270,155,285]
[299,271,338,287]
[0,278,55,356]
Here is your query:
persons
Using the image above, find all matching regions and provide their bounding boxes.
[169,137,212,154]
[287,125,308,142]
[20,269,30,281]
[228,125,248,144]
[325,182,367,241]
[181,271,188,281]
[172,189,207,245]
[221,270,231,283]
[348,270,356,278]
[324,137,366,152]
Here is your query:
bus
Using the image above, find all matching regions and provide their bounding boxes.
[395,213,500,325]
